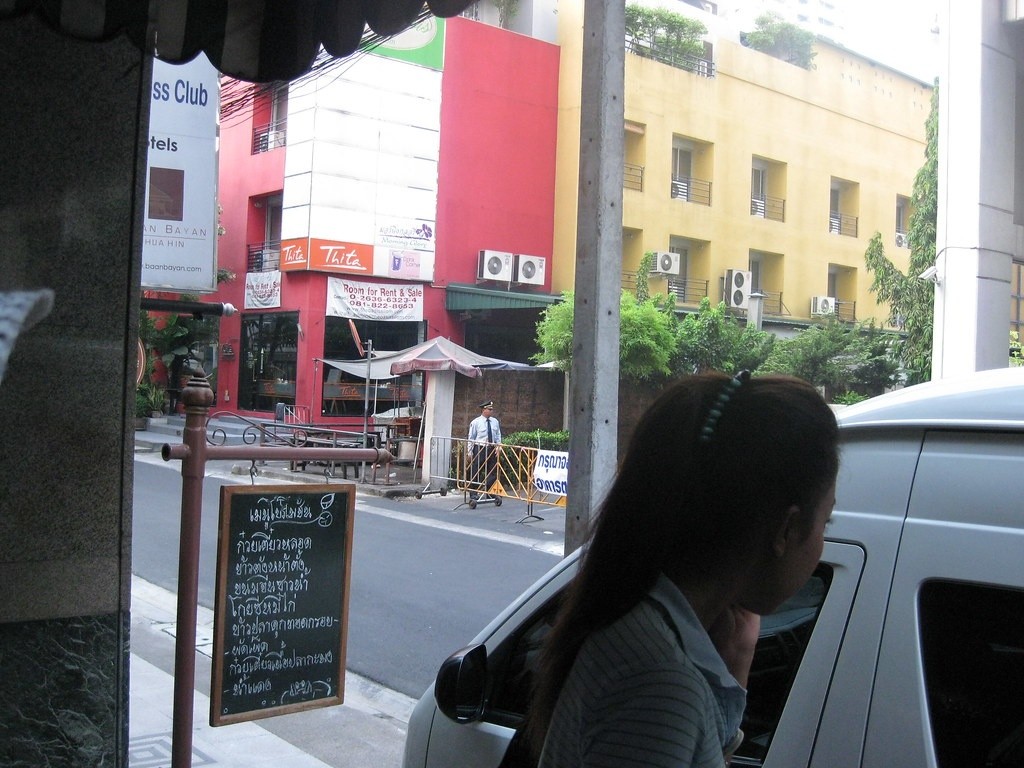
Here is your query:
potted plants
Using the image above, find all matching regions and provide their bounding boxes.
[135,385,167,430]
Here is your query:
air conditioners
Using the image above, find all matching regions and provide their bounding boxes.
[829,218,839,234]
[260,130,284,153]
[512,254,545,286]
[810,297,835,318]
[751,199,765,217]
[648,252,680,275]
[252,249,279,272]
[896,233,909,249]
[671,180,688,201]
[723,269,752,309]
[476,249,513,282]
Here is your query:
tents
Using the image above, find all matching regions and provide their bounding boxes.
[310,336,540,424]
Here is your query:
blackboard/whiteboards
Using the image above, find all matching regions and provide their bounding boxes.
[209,483,356,729]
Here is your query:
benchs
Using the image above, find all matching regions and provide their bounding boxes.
[287,437,359,479]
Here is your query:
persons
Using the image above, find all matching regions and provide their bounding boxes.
[468,400,502,500]
[500,369,842,768]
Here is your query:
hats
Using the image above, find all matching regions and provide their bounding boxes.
[479,400,495,409]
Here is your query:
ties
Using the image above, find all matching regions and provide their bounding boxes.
[486,419,493,443]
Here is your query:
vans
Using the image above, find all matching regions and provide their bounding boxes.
[402,368,1024,768]
